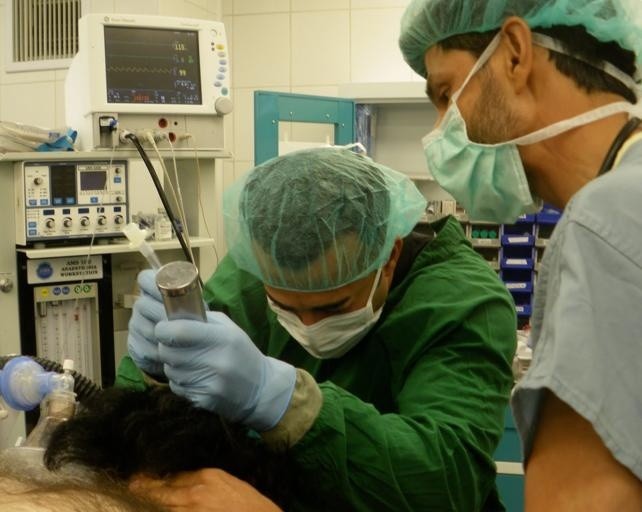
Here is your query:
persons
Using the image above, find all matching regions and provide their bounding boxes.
[127,0,642,512]
[124,144,519,512]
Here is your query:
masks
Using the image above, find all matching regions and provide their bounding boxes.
[421,103,544,224]
[267,296,385,360]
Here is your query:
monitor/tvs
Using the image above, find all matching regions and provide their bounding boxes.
[87,12,231,113]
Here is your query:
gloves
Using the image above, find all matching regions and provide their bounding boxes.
[127,269,296,434]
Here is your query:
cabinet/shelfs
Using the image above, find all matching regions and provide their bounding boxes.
[459,203,561,319]
[1,144,232,261]
